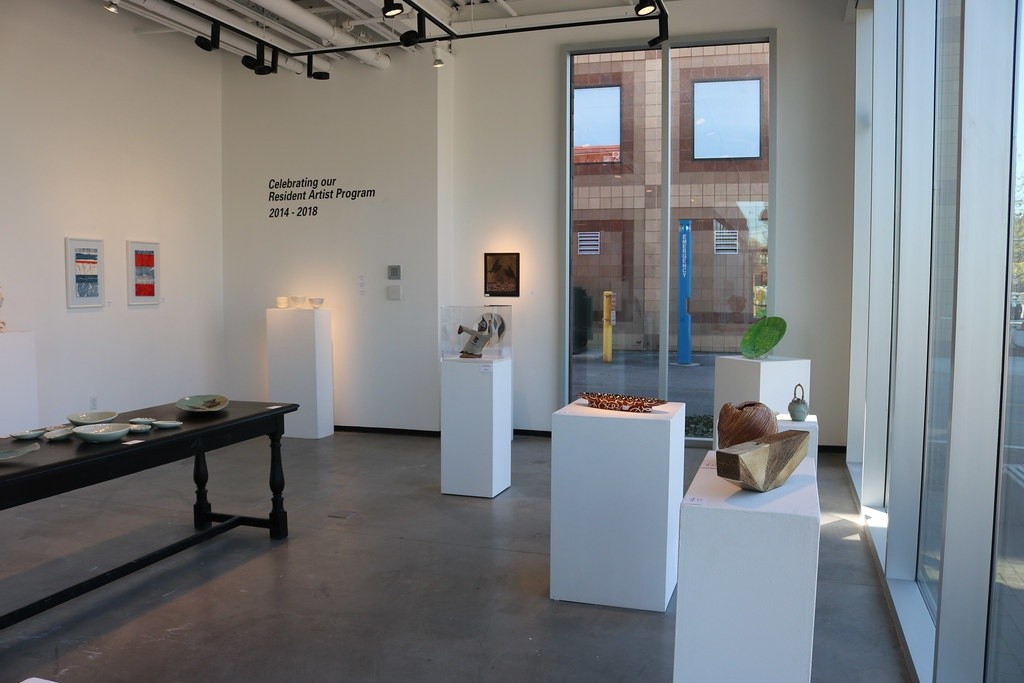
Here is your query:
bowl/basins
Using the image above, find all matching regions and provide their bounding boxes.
[66,412,119,424]
[74,422,131,443]
[276,296,325,309]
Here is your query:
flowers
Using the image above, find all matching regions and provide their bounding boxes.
[484,253,520,296]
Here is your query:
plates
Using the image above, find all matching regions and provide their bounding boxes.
[130,418,184,433]
[576,391,670,412]
[176,393,227,413]
[9,424,73,440]
[740,316,787,357]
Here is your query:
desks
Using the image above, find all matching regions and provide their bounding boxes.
[0,400,299,628]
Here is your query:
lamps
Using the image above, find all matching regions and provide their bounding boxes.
[104,0,656,80]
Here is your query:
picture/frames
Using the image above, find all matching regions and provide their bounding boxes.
[126,241,161,305]
[64,238,106,308]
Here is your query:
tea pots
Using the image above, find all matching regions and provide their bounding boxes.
[789,384,808,421]
